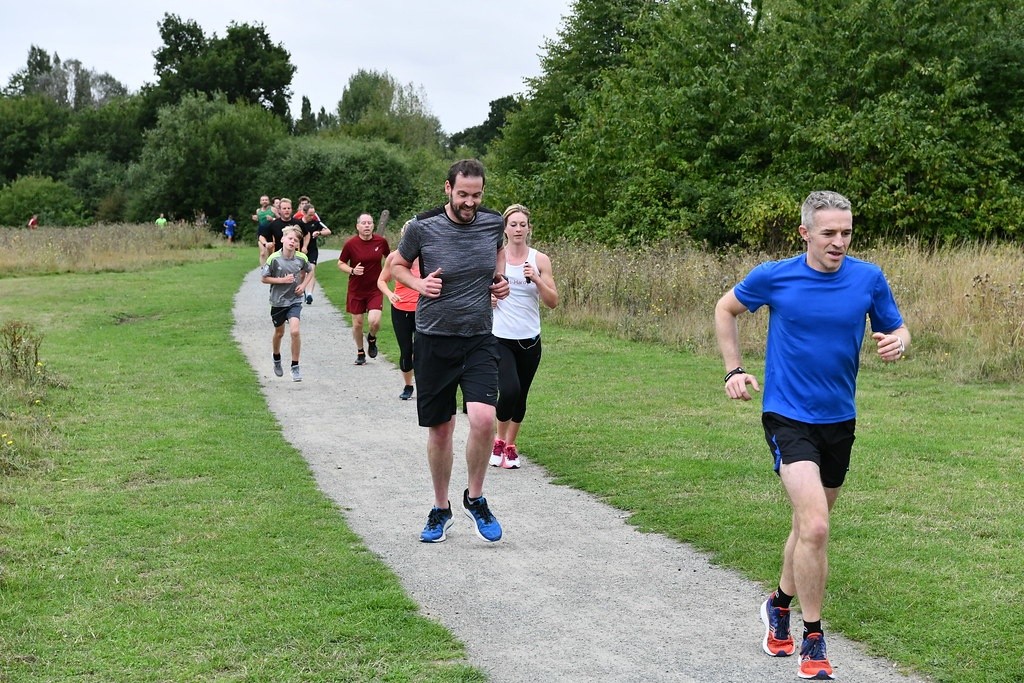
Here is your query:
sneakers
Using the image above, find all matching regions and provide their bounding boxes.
[272,356,284,377]
[463,489,502,541]
[419,499,454,542]
[355,351,366,365]
[290,365,303,382]
[367,336,378,358]
[797,632,836,679]
[400,384,414,399]
[760,590,795,656]
[502,445,521,469]
[488,437,507,466]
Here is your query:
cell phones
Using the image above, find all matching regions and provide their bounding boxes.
[525,262,531,284]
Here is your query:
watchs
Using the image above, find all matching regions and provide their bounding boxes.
[898,338,904,352]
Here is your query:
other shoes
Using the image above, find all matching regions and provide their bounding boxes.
[306,295,313,304]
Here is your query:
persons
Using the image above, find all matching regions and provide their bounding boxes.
[29,215,38,229]
[715,191,911,678]
[262,224,314,381]
[252,195,331,304]
[392,159,510,543]
[224,215,236,241]
[489,203,557,469]
[338,214,390,365]
[155,213,167,228]
[377,220,421,399]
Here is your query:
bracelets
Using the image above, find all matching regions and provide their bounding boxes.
[502,275,508,281]
[351,269,354,275]
[725,367,745,382]
[319,231,321,235]
